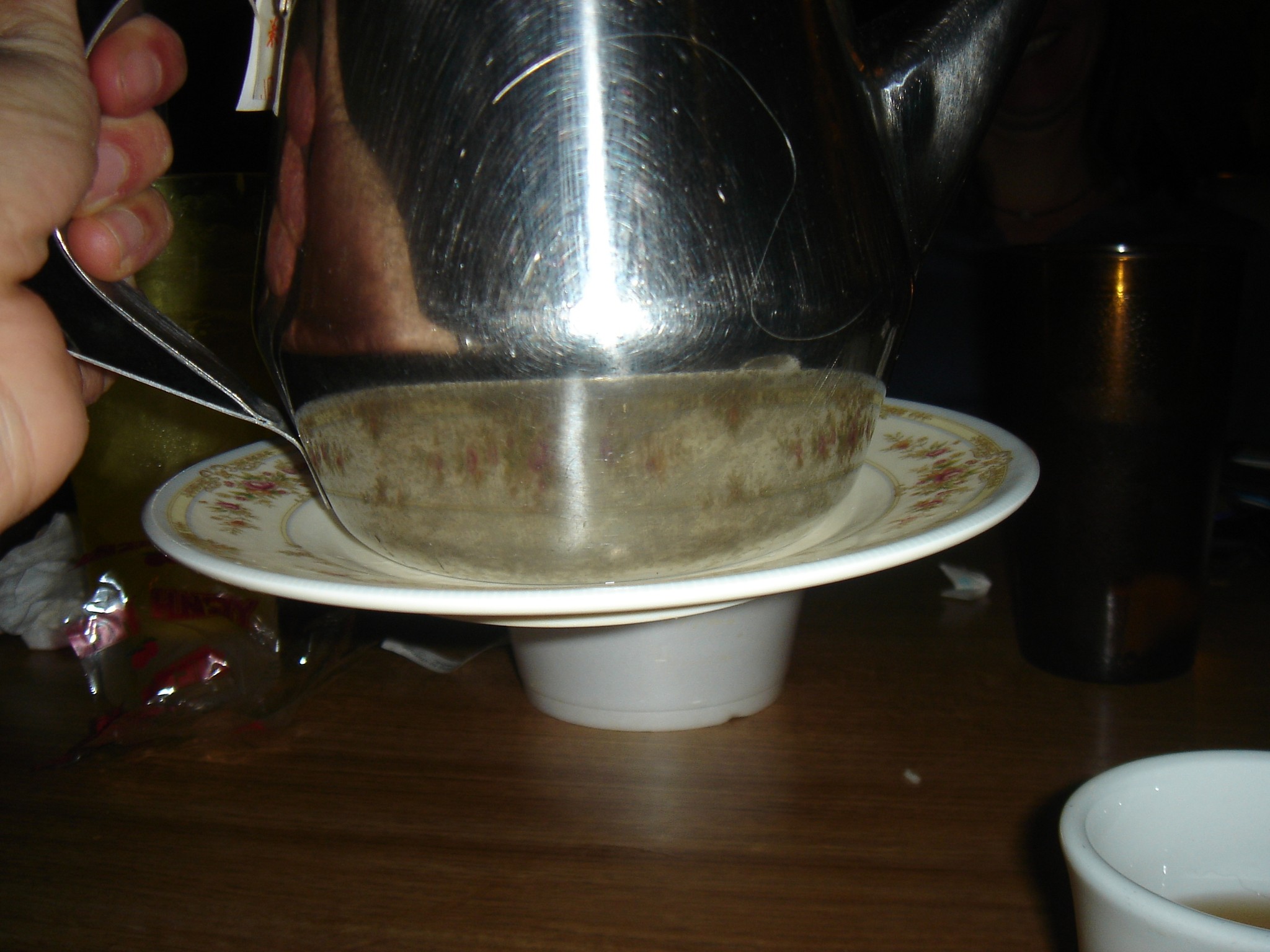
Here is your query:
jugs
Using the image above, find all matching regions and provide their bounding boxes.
[25,0,1019,585]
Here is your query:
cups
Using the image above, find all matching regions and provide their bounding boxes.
[507,591,801,731]
[1059,748,1270,952]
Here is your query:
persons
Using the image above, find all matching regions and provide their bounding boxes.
[264,0,471,357]
[0,0,186,538]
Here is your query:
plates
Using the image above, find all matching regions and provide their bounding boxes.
[146,392,1044,631]
